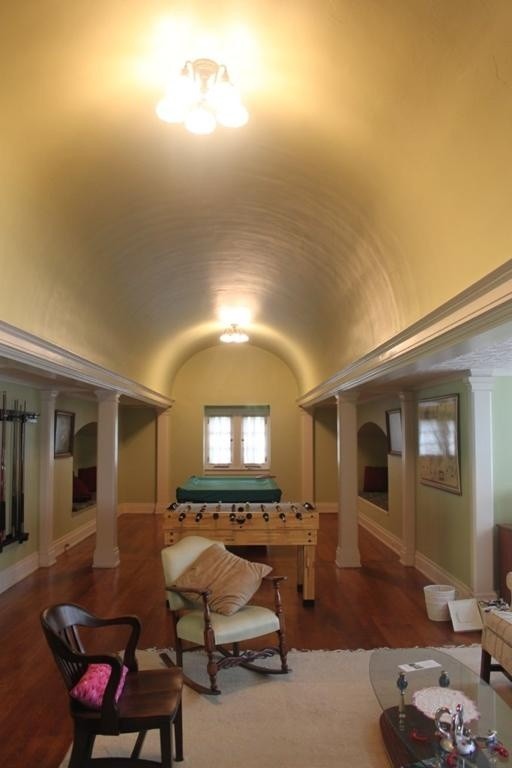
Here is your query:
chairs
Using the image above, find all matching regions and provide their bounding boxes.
[41,603,186,767]
[159,544,292,696]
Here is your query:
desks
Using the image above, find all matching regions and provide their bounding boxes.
[161,474,322,610]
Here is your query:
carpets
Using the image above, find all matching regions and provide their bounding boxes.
[56,645,511,767]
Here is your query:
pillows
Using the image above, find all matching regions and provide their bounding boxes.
[166,544,274,618]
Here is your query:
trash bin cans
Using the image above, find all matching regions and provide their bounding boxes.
[423,583,457,623]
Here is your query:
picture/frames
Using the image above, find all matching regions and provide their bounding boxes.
[52,409,74,461]
[384,391,463,497]
